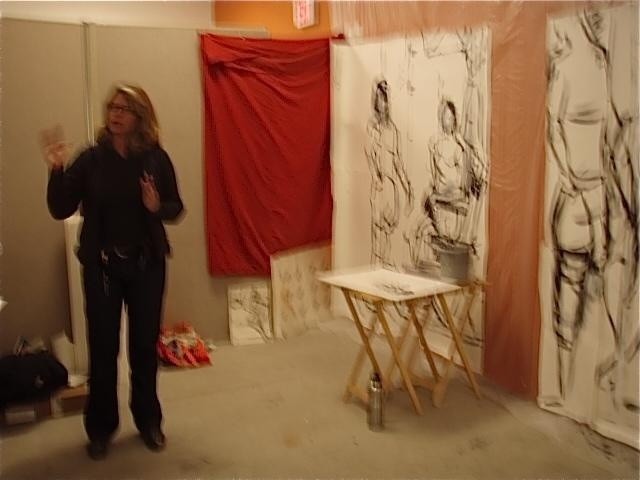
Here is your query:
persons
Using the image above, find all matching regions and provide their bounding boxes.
[38,85,185,459]
[545,10,639,416]
[364,74,414,270]
[423,99,481,245]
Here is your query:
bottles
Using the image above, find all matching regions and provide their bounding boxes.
[367,374,385,432]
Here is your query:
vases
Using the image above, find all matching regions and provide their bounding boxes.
[431,245,470,287]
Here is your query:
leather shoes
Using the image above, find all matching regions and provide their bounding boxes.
[141,428,166,452]
[87,439,109,458]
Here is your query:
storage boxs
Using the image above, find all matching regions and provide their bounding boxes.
[1,398,37,426]
[50,381,89,418]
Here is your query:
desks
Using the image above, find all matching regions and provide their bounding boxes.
[317,266,465,415]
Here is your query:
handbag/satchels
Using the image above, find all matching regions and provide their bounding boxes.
[0,335,68,401]
